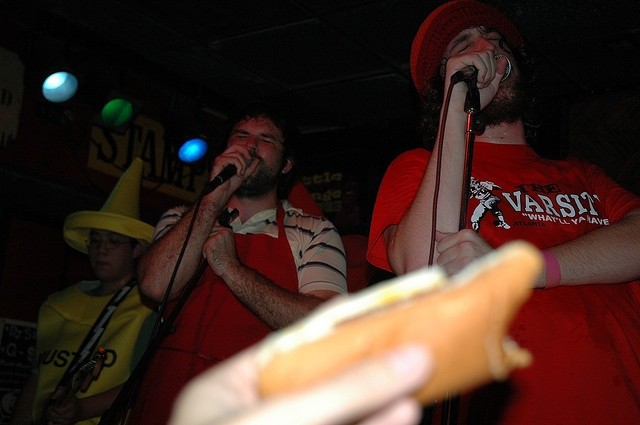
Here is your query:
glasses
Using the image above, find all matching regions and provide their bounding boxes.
[85,238,133,252]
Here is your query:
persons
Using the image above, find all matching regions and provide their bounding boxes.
[365,1,640,425]
[122,100,350,425]
[11,226,164,424]
[169,291,433,425]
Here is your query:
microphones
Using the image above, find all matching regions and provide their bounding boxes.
[201,149,254,193]
[448,50,513,85]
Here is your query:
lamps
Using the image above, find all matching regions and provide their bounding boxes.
[92,80,133,127]
[164,118,208,166]
[27,53,79,103]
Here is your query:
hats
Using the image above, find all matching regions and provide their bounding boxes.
[410,0,521,104]
[63,156,158,252]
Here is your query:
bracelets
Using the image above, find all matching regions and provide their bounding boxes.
[540,250,560,289]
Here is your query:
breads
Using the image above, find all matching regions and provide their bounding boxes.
[254,239,544,408]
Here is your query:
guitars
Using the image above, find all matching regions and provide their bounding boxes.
[35,351,107,425]
